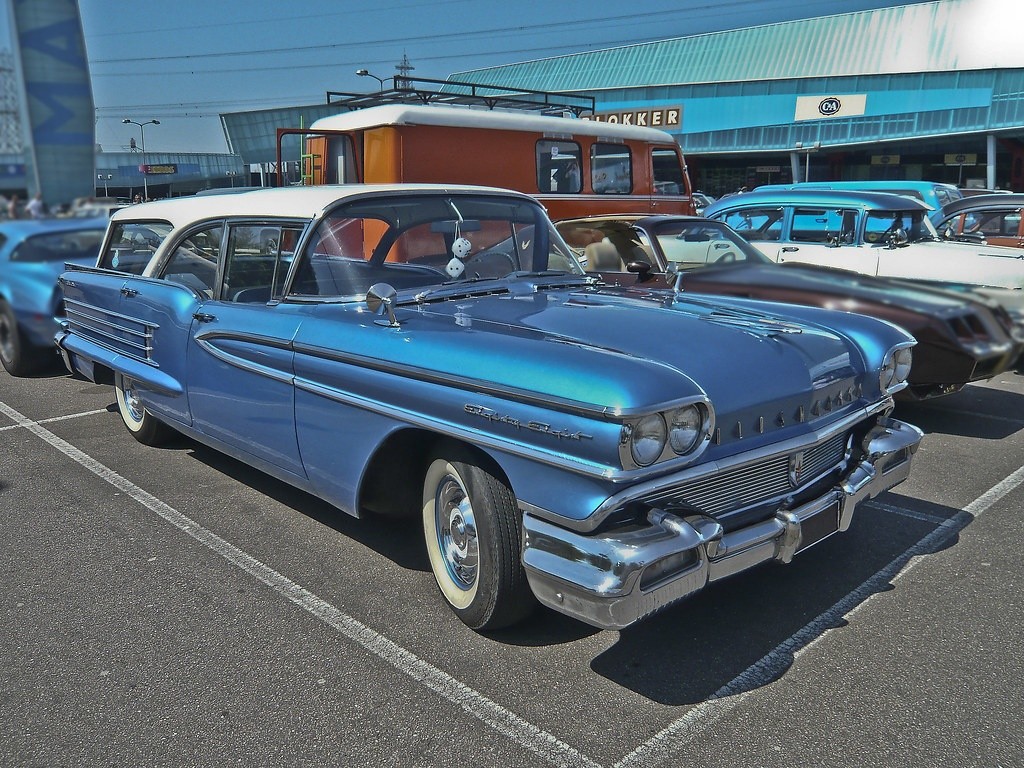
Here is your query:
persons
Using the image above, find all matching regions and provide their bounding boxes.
[738,187,747,194]
[6,195,19,219]
[84,196,95,208]
[22,192,46,220]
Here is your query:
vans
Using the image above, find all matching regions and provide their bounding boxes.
[753,180,976,229]
[275,102,698,264]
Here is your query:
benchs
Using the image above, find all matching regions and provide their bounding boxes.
[232,276,439,303]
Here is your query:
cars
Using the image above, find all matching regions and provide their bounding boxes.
[403,211,1024,402]
[602,189,1024,314]
[916,193,1023,244]
[957,188,1013,198]
[1,211,114,380]
[52,183,924,641]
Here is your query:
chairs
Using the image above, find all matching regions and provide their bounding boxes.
[630,246,662,274]
[585,240,626,272]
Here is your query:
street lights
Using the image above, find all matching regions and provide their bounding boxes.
[355,69,395,92]
[122,118,160,199]
